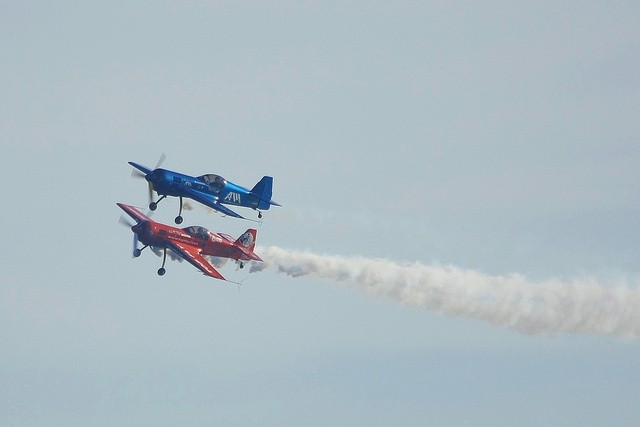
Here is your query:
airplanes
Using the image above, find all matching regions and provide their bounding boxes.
[128,161,283,224]
[116,201,264,280]
